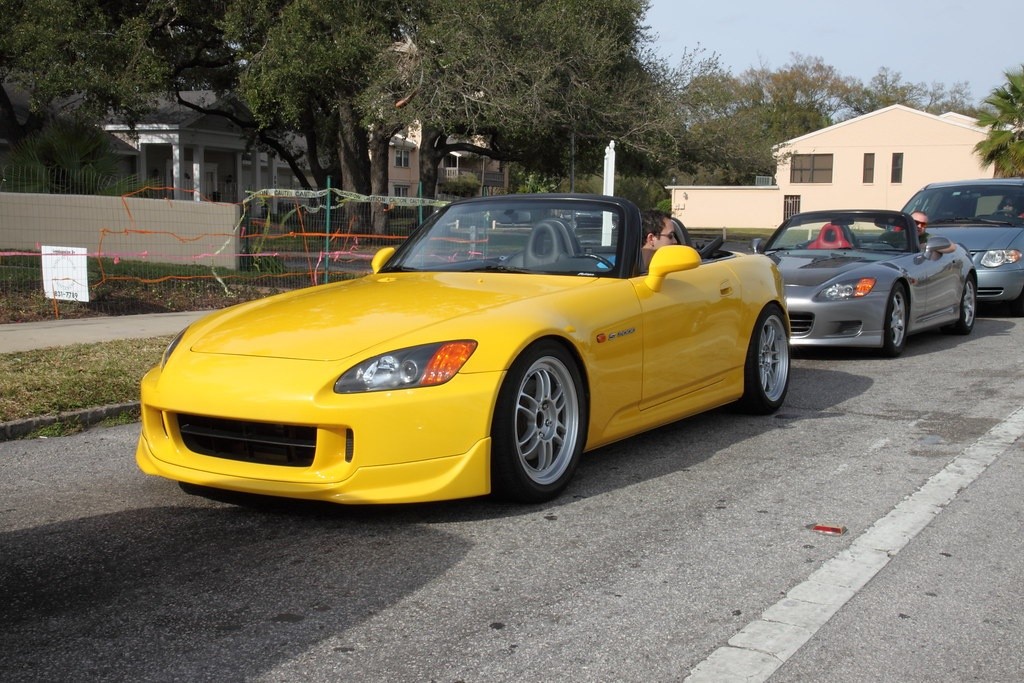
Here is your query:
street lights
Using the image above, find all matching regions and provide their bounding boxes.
[567,101,585,230]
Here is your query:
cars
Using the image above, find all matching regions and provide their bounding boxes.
[749,209,978,358]
[136,192,791,504]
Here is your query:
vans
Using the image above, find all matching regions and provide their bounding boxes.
[875,177,1024,302]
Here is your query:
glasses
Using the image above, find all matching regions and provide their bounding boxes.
[914,220,927,227]
[655,232,673,239]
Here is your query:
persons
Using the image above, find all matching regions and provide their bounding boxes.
[596,209,678,274]
[876,209,957,254]
[992,196,1024,219]
